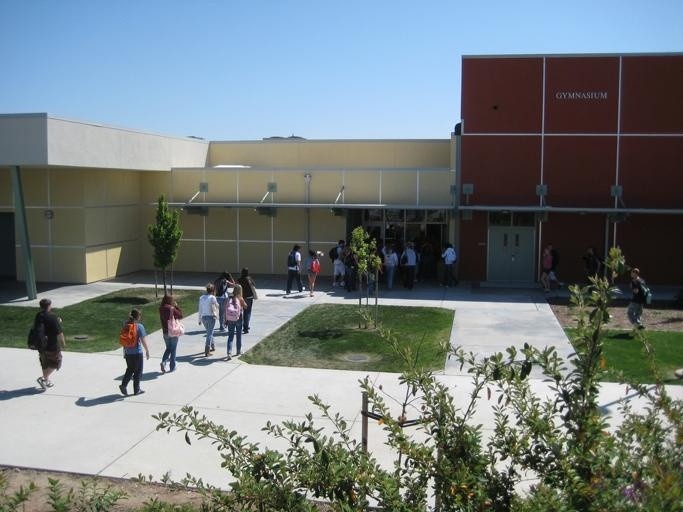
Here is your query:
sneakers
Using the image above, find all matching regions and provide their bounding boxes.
[37,327,251,396]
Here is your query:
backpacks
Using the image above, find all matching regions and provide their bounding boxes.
[345,253,353,267]
[117,324,138,347]
[329,247,338,261]
[225,297,241,321]
[638,285,651,305]
[288,251,296,266]
[28,322,48,350]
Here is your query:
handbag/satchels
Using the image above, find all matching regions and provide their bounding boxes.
[312,257,321,273]
[167,318,184,337]
[401,256,407,264]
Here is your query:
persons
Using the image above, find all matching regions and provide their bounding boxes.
[541,248,553,293]
[578,247,601,295]
[198,267,258,360]
[119,309,150,395]
[627,268,648,332]
[441,243,459,287]
[159,296,184,373]
[29,299,65,390]
[286,239,418,297]
[545,243,564,289]
